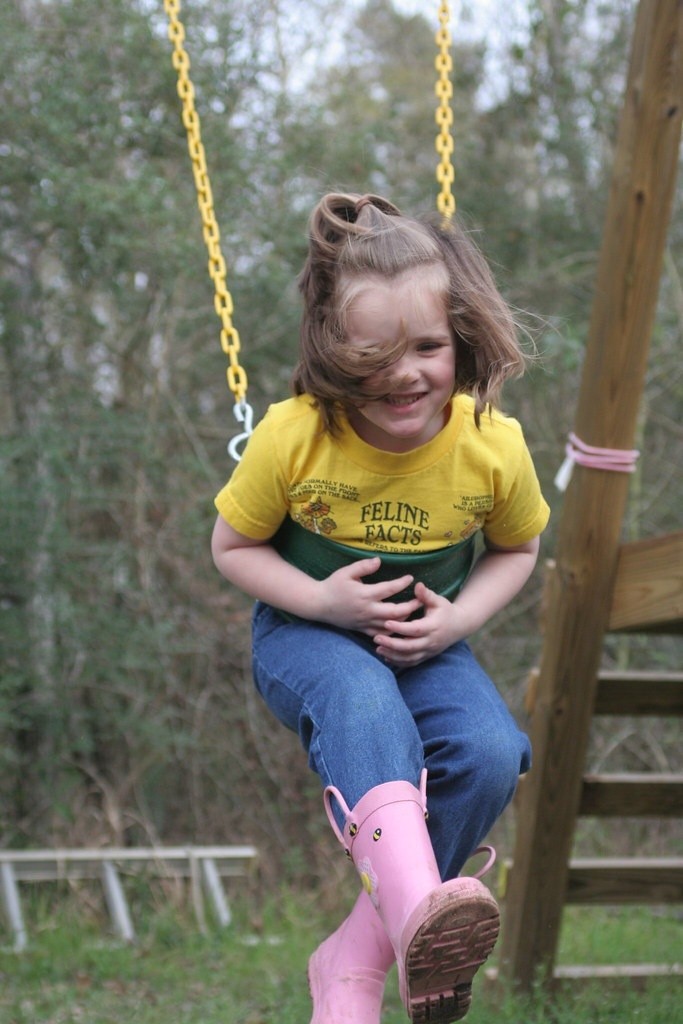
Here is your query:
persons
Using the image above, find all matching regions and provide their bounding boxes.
[212,191,551,1024]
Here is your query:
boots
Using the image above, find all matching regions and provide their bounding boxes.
[323,769,499,1024]
[308,846,495,1024]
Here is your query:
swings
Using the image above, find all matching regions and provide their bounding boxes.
[160,0,481,661]
